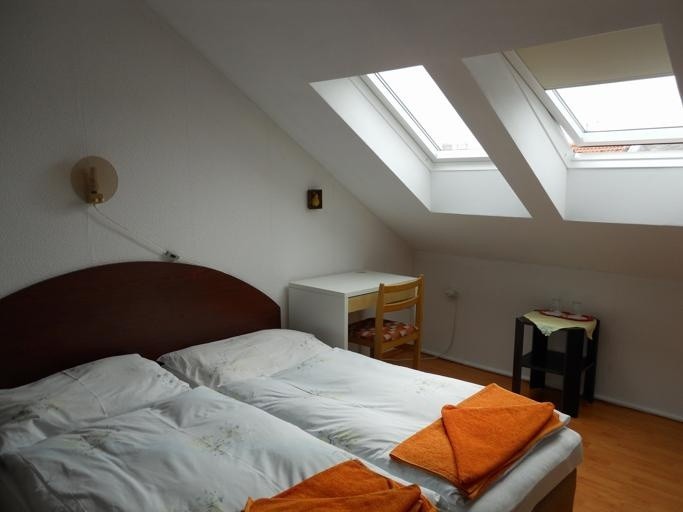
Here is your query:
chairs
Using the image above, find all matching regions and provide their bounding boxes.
[347,273,426,370]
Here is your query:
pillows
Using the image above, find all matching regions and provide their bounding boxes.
[1,352,192,456]
[158,327,332,390]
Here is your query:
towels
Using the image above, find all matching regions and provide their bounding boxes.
[245,459,438,512]
[390,381,567,501]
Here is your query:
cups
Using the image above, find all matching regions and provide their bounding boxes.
[571,301,582,316]
[552,298,560,312]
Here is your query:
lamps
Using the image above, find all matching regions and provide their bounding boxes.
[72,156,119,205]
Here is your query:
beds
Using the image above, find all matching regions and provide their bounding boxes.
[0,261,582,512]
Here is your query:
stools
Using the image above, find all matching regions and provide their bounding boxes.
[512,308,600,418]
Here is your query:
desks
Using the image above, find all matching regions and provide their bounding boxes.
[289,269,420,351]
